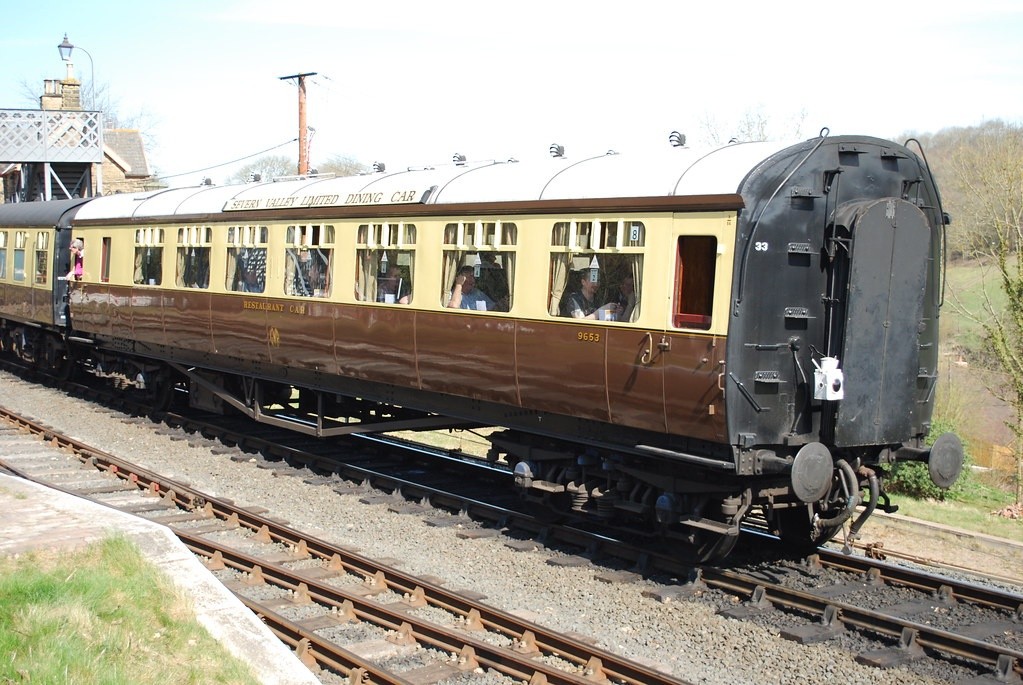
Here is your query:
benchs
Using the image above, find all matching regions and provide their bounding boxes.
[453,267,508,308]
[377,265,411,295]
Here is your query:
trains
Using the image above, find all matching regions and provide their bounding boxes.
[0,124,964,569]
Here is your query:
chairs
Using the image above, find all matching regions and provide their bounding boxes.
[557,264,632,310]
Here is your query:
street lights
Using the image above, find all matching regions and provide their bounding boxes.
[57,32,95,113]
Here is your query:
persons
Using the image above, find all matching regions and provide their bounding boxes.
[68,239,84,259]
[562,268,624,321]
[376,264,411,305]
[605,273,634,309]
[477,250,507,300]
[448,266,498,312]
